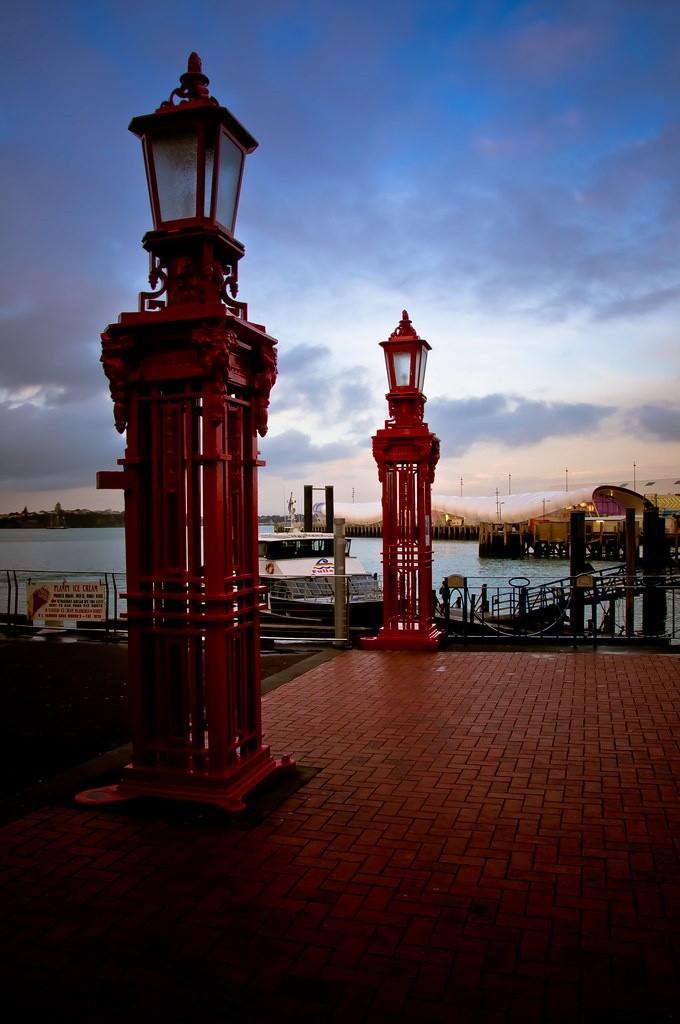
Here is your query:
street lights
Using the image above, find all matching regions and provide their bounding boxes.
[75,49,295,816]
[368,310,444,651]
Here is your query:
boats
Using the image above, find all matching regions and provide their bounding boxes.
[260,484,385,626]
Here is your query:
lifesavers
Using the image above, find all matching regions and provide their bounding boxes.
[267,563,274,574]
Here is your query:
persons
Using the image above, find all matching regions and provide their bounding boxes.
[281,540,333,556]
[439,581,450,614]
